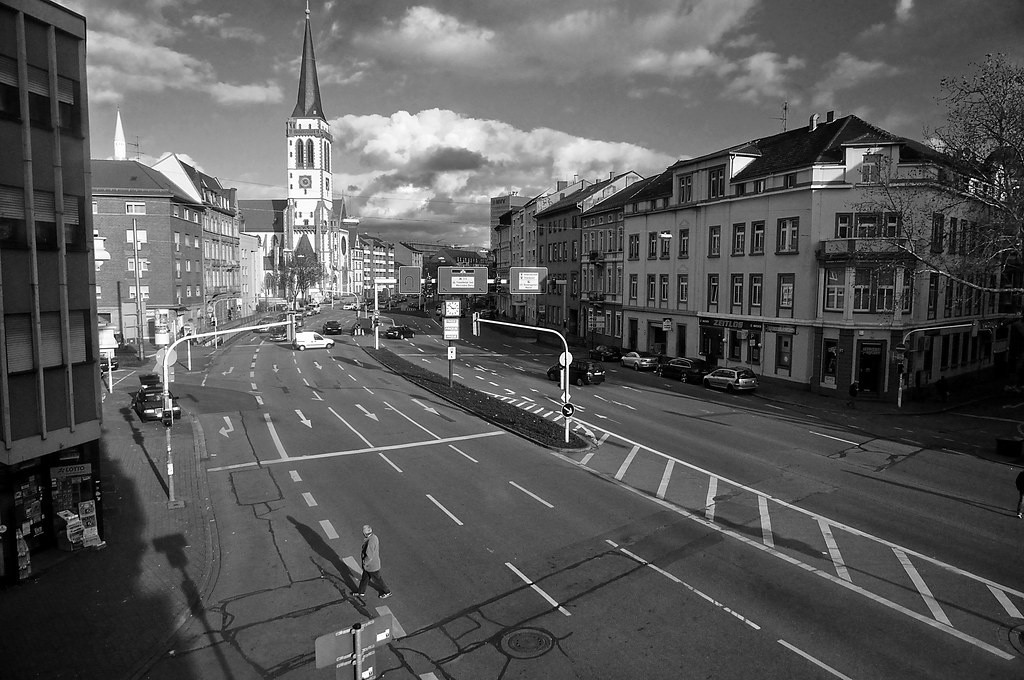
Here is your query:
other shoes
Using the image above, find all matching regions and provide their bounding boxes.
[852,408,856,409]
[846,403,850,407]
[379,591,392,599]
[353,593,365,596]
[1017,513,1023,518]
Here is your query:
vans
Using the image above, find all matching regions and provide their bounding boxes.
[307,303,321,314]
[294,332,336,351]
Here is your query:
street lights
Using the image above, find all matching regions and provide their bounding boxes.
[555,279,568,338]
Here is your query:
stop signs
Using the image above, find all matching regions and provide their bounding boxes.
[895,343,907,354]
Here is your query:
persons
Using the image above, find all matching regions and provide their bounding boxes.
[351,525,392,598]
[847,380,860,407]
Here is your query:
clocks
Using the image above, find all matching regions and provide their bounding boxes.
[298,175,313,189]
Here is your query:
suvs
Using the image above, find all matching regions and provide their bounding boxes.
[547,358,605,387]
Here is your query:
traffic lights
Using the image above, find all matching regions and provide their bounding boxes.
[898,363,904,373]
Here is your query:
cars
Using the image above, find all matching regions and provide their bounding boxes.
[655,356,707,383]
[133,374,182,422]
[322,320,342,335]
[342,293,407,312]
[619,350,658,372]
[435,304,442,316]
[267,304,288,311]
[588,345,623,362]
[252,308,307,342]
[702,364,760,394]
[385,324,415,340]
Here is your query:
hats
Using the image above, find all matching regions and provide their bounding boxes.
[855,380,859,383]
